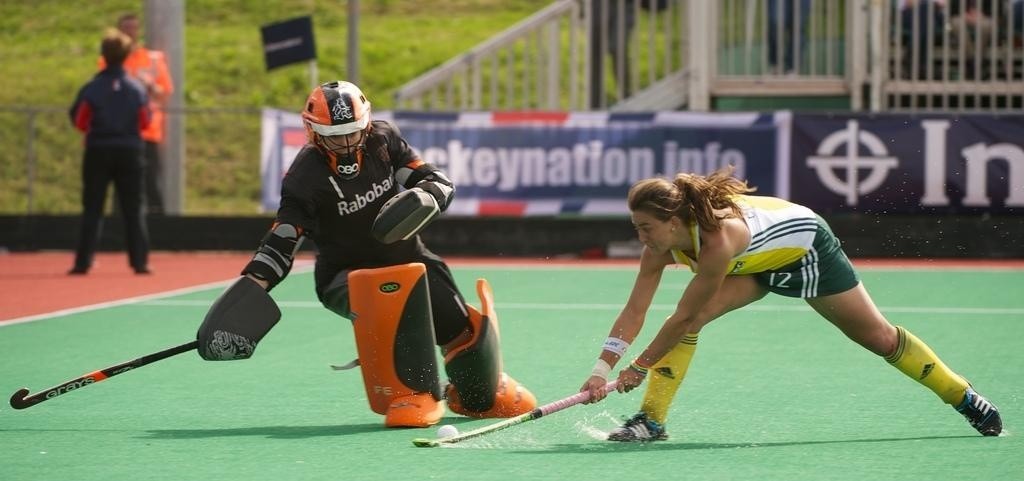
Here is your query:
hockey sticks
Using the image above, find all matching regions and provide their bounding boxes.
[411,376,620,446]
[10,339,201,409]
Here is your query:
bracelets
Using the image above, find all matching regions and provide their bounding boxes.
[588,358,611,384]
[629,360,655,378]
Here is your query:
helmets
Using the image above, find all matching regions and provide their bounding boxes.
[303,81,372,180]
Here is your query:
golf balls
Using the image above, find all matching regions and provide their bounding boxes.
[438,424,458,440]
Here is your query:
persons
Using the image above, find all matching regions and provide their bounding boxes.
[580,165,1001,440]
[767,0,811,76]
[198,82,537,427]
[69,31,150,275]
[582,0,634,110]
[97,15,174,217]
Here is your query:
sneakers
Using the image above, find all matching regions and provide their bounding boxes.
[608,410,668,441]
[953,385,1003,436]
